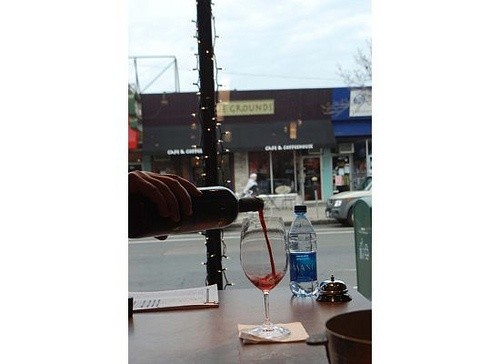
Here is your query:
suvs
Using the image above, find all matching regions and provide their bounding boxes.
[325,175,372,226]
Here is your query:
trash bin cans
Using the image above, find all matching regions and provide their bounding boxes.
[352,196,372,301]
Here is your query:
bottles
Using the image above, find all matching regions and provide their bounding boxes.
[128,186,264,238]
[288,205,319,298]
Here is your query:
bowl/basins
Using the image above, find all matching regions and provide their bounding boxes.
[324,309,371,363]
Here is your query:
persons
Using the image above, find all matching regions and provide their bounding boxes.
[129,169,204,241]
[243,172,258,196]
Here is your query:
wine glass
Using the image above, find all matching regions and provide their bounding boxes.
[240,216,290,340]
